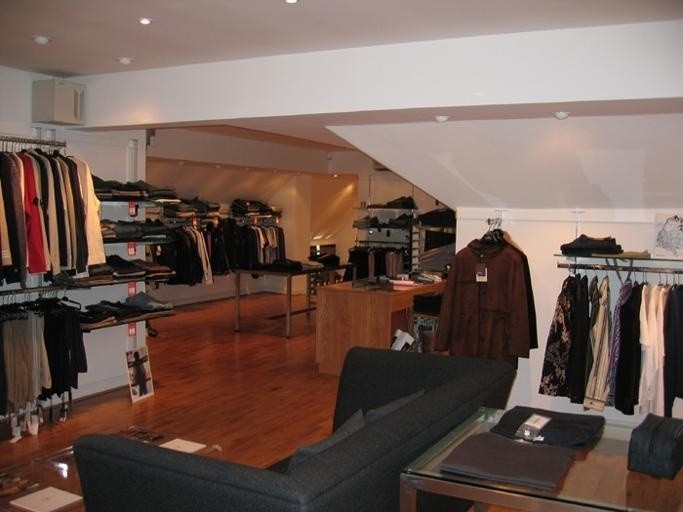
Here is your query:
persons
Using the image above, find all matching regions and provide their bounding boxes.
[132,352,147,397]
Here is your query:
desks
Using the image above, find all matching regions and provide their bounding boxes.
[316,273,449,375]
[400,404,681,510]
[234,261,330,340]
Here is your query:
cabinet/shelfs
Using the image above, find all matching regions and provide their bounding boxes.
[65,190,176,331]
[358,203,417,247]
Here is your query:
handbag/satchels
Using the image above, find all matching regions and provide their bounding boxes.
[626,413,683,481]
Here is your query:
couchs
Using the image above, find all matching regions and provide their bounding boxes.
[72,342,517,509]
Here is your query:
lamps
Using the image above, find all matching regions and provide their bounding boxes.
[554,111,572,120]
[115,55,135,66]
[434,115,449,123]
[31,35,52,46]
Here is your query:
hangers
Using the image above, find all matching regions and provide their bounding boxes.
[1,286,65,309]
[486,219,503,230]
[0,137,67,155]
[156,214,280,227]
[558,262,683,288]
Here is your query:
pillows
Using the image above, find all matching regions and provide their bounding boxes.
[366,387,424,423]
[287,407,366,472]
